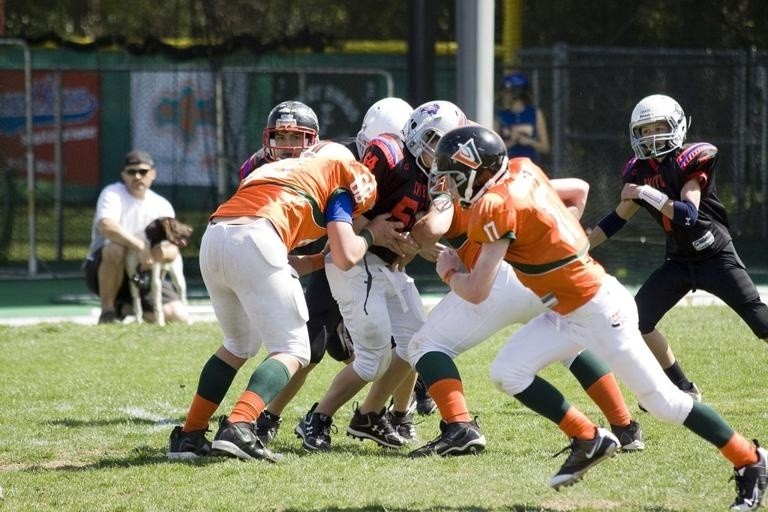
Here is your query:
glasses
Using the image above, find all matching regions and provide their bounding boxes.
[124,167,150,175]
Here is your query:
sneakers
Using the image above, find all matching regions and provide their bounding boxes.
[256,409,283,448]
[638,381,702,415]
[344,400,404,449]
[168,424,212,463]
[387,398,423,448]
[293,400,335,452]
[211,414,284,465]
[115,304,126,322]
[610,419,645,451]
[98,309,122,326]
[416,391,439,417]
[406,416,487,458]
[726,437,767,512]
[548,427,623,488]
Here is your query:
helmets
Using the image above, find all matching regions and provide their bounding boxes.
[352,97,510,214]
[628,92,688,162]
[262,100,320,163]
[498,73,530,93]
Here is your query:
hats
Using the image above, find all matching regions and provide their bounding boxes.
[121,149,156,170]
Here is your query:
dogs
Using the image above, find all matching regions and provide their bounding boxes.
[125,217,193,327]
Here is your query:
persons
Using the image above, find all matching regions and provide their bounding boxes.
[168,98,644,463]
[81,149,177,320]
[588,94,767,412]
[493,74,551,166]
[430,126,768,511]
